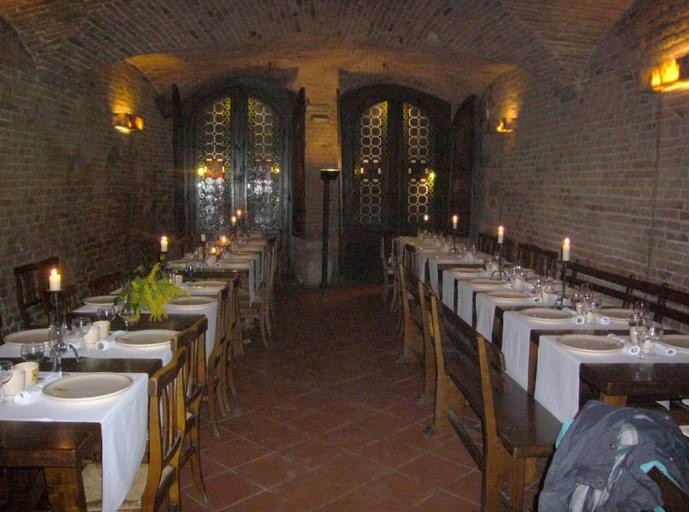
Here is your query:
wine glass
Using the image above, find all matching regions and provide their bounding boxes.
[70,316,91,351]
[20,341,44,362]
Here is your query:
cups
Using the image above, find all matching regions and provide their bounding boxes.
[14,362,39,388]
[83,327,99,345]
[3,370,25,396]
[92,320,110,339]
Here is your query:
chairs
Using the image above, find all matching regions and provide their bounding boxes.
[375,229,689,511]
[0,229,293,511]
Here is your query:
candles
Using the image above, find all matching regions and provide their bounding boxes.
[497,225,504,242]
[452,215,458,232]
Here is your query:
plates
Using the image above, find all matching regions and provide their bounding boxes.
[2,228,269,401]
[421,228,689,356]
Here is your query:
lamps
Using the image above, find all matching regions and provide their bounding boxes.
[113,112,144,134]
[637,55,689,94]
[319,167,340,181]
[497,118,517,136]
